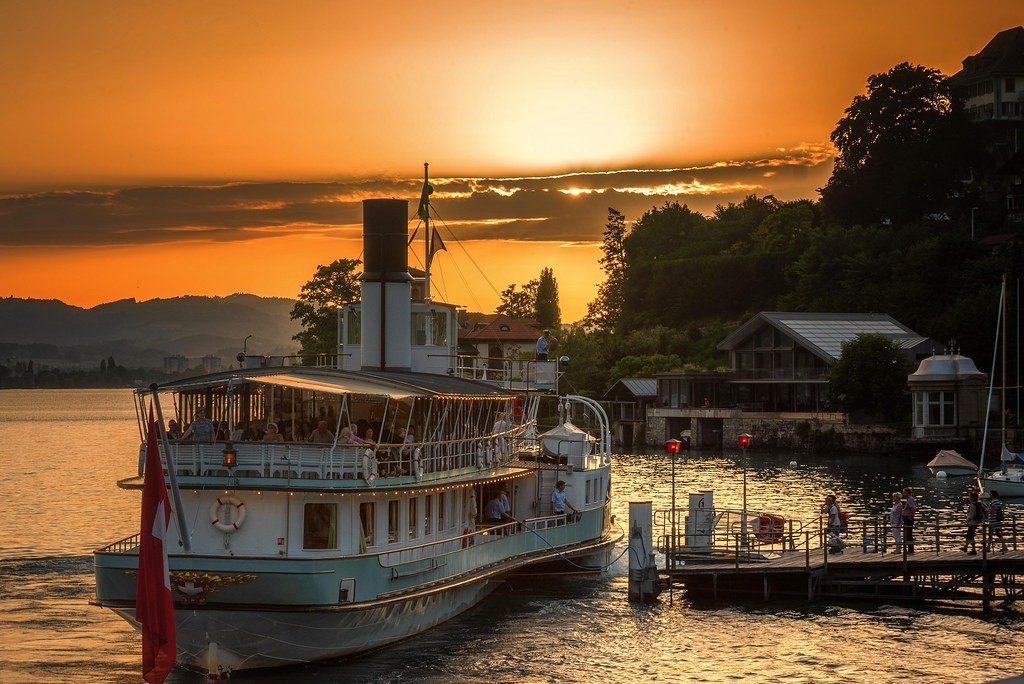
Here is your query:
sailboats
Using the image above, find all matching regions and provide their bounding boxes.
[974,272,1024,500]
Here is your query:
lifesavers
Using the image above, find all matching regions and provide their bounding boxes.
[363,448,377,486]
[413,447,424,480]
[495,439,502,463]
[486,441,493,467]
[210,497,247,531]
[477,443,485,472]
[502,438,508,458]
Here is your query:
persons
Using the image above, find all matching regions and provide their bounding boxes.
[890,487,917,554]
[536,329,551,361]
[959,490,1009,556]
[550,480,580,526]
[166,407,417,478]
[824,493,842,556]
[485,482,511,537]
[493,412,516,433]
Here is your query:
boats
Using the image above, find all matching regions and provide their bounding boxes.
[84,198,629,684]
[926,450,979,478]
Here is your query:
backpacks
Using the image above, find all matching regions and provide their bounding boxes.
[971,502,984,523]
[994,501,1003,521]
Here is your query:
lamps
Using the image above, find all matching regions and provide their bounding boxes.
[221,443,238,471]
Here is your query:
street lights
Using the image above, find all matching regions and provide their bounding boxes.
[736,432,752,549]
[664,438,682,568]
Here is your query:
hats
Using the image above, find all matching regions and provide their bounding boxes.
[893,492,902,501]
[267,423,278,434]
[543,330,551,333]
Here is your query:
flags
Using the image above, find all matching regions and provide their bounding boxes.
[135,397,178,684]
[428,227,446,268]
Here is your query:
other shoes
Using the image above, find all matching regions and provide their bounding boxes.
[967,551,977,555]
[381,468,407,478]
[981,548,991,552]
[999,548,1007,552]
[959,548,967,552]
[891,550,901,554]
